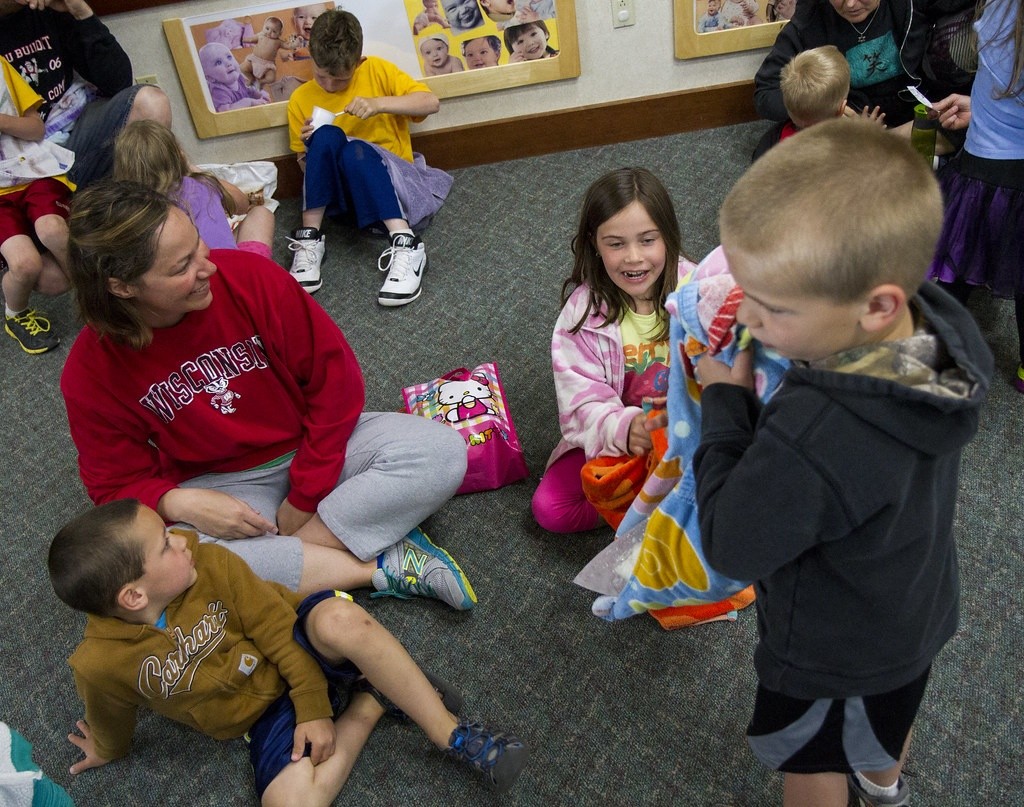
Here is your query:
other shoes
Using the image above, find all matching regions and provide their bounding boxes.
[847,773,910,807]
[1015,363,1024,391]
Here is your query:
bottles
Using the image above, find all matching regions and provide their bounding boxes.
[910,104,936,169]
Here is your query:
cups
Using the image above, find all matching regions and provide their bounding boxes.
[306,105,336,140]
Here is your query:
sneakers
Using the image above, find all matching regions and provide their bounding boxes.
[5,306,60,354]
[369,526,478,611]
[285,227,327,293]
[377,233,427,306]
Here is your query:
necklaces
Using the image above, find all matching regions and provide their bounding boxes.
[848,2,881,44]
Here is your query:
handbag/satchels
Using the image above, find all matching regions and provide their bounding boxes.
[401,362,530,496]
[197,160,279,231]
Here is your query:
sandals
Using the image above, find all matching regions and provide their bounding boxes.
[352,668,463,721]
[438,716,529,793]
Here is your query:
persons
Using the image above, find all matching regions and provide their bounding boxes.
[751,45,888,166]
[531,164,700,535]
[752,0,983,131]
[928,0,1024,396]
[287,10,440,308]
[58,181,479,612]
[0,0,275,356]
[188,2,327,113]
[697,114,994,807]
[48,498,530,807]
[403,0,560,76]
[697,0,764,32]
